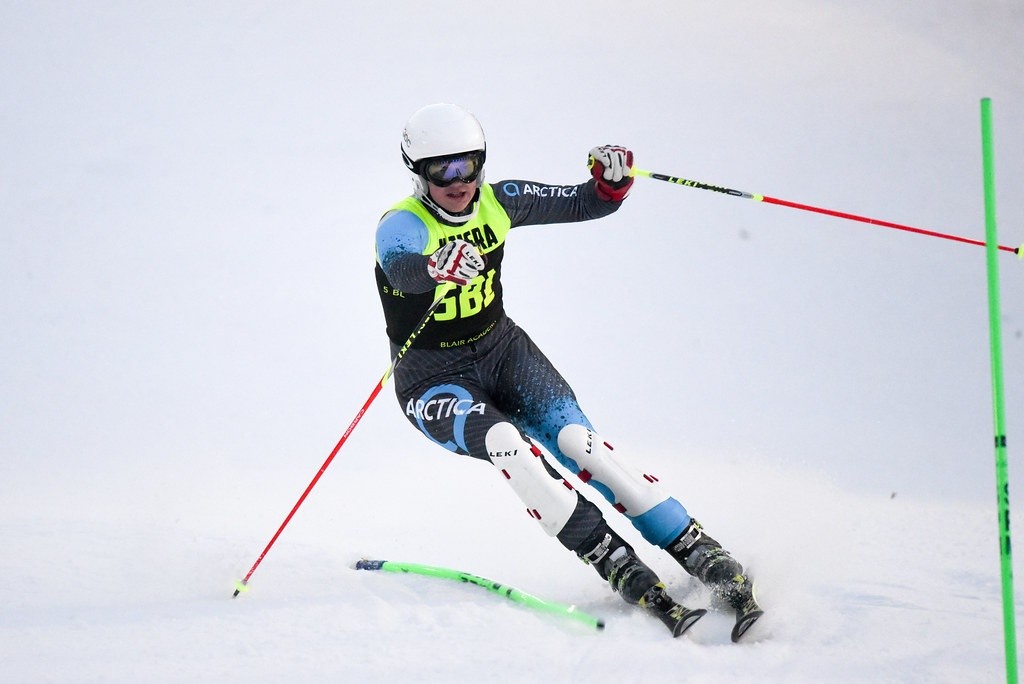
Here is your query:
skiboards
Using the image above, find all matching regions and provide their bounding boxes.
[649,585,765,645]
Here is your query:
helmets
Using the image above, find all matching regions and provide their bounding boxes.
[400,103,487,163]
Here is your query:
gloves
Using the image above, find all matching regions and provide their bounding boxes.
[587,145,640,201]
[426,238,486,287]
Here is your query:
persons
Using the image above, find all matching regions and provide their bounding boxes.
[374,105,754,612]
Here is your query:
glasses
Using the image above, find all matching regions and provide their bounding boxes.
[400,142,487,188]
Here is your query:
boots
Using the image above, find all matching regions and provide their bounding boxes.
[555,494,667,607]
[666,519,743,588]
